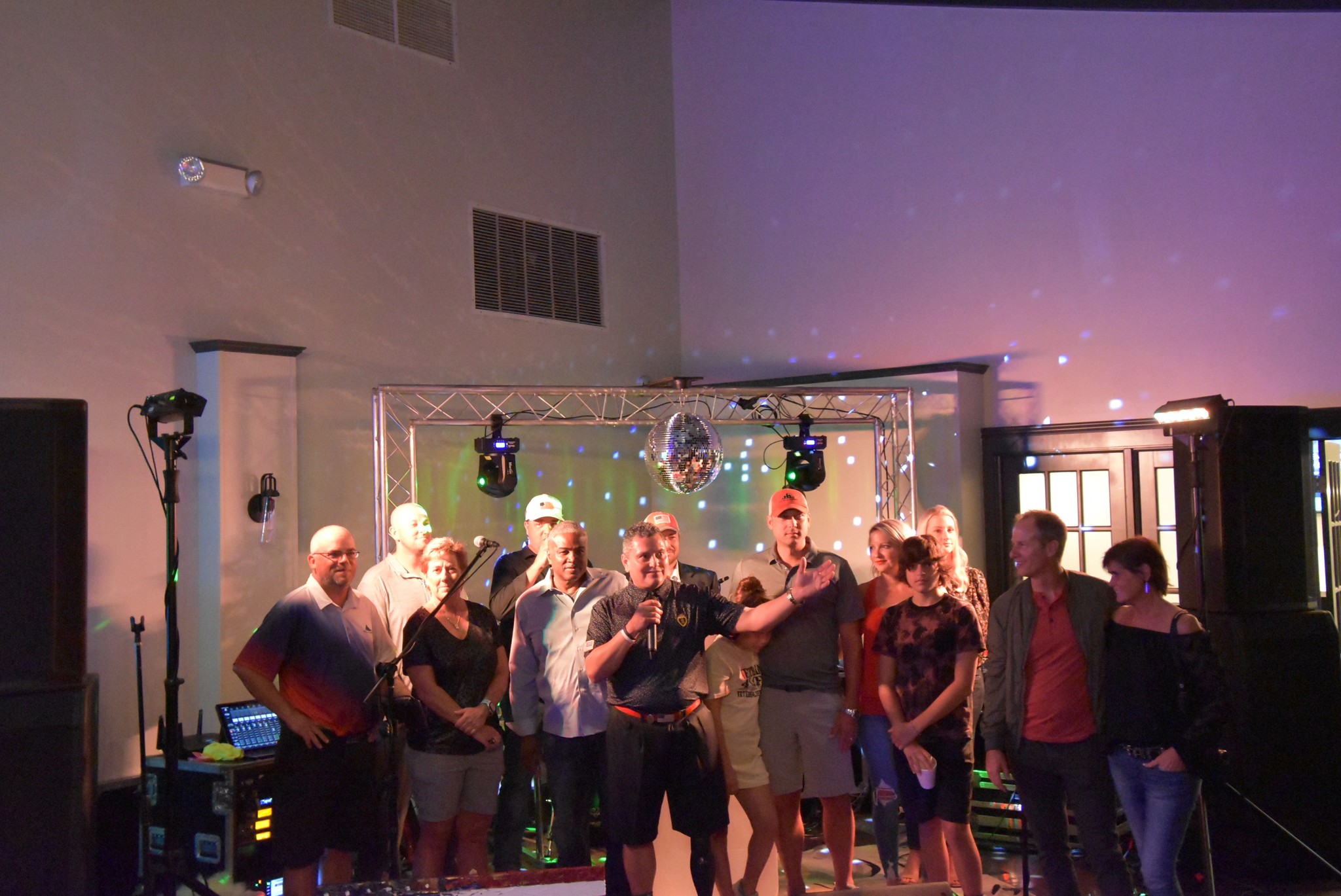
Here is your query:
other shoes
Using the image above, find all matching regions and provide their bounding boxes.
[900,865,921,883]
[948,866,961,887]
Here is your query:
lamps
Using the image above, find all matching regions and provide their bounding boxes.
[248,473,279,545]
[140,388,208,460]
[474,414,520,498]
[1153,394,1227,436]
[783,414,827,492]
[644,396,724,494]
[178,156,263,198]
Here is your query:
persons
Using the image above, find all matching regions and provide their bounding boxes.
[357,503,468,870]
[1099,536,1231,896]
[980,513,1135,896]
[402,536,509,884]
[232,523,397,896]
[488,488,991,896]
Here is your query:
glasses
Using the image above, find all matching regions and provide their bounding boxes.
[311,548,359,559]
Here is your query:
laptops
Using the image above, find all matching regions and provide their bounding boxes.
[215,699,283,760]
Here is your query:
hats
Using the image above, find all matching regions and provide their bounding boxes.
[769,489,808,518]
[525,493,564,522]
[644,511,680,534]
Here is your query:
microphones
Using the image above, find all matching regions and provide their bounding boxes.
[474,535,499,548]
[645,596,659,659]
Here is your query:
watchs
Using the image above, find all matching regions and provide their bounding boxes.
[479,699,496,717]
[840,707,861,721]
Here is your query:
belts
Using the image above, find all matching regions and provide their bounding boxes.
[613,700,701,724]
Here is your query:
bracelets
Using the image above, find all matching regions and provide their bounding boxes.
[621,624,636,644]
[787,587,806,608]
[910,719,922,736]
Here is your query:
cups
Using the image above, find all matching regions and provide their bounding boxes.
[915,757,937,789]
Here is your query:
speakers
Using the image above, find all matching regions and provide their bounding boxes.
[1171,405,1321,613]
[0,676,100,896]
[1180,607,1341,896]
[0,397,86,689]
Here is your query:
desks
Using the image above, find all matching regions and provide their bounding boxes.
[138,754,277,878]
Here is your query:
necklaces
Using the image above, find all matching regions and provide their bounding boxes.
[432,600,461,631]
